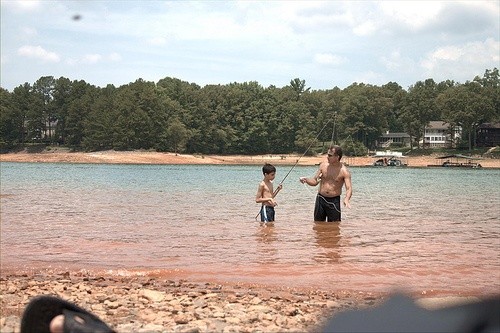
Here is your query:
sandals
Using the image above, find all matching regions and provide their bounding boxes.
[19,295,115,333]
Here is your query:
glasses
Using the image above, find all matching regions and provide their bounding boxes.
[327,154,334,157]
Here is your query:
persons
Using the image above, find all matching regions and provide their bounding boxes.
[255,163,283,225]
[299,145,353,223]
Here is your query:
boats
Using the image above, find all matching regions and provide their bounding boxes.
[372,149,409,167]
[427,154,483,169]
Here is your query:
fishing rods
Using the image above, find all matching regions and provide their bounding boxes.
[255,113,336,220]
